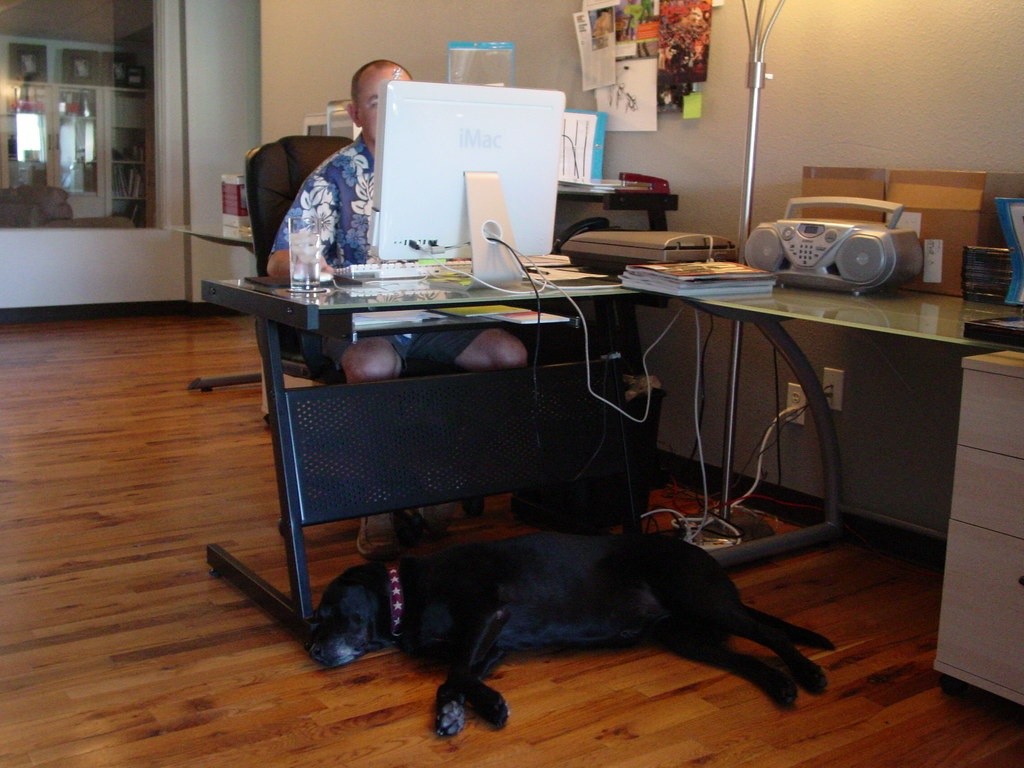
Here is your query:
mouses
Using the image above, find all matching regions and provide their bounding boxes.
[318,272,334,283]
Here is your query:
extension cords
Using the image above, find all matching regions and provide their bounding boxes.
[691,537,734,553]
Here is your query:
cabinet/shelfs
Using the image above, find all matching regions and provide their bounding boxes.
[934,350,1024,706]
[0,44,147,220]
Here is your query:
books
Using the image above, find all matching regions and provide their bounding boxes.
[617,262,778,297]
[111,164,141,198]
[130,205,138,222]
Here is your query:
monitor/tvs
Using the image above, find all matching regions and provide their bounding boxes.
[369,80,565,291]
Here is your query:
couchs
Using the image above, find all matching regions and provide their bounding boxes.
[0,184,135,228]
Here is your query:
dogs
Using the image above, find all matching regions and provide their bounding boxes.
[592,12,612,49]
[302,529,838,739]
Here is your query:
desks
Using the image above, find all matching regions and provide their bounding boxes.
[163,223,262,391]
[553,181,676,232]
[703,285,1024,583]
[202,267,644,646]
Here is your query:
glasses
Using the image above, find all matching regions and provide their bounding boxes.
[353,96,379,113]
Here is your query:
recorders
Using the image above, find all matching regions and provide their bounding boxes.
[745,218,923,297]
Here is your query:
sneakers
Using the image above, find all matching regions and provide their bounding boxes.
[420,501,456,538]
[356,510,398,561]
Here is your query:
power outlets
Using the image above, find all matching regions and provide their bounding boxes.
[785,383,805,425]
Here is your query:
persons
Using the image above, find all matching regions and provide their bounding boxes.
[669,36,687,71]
[267,60,568,554]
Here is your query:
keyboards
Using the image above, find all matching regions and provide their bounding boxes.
[335,260,473,279]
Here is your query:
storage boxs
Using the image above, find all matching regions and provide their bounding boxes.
[886,169,986,298]
[220,174,250,227]
[802,166,886,221]
